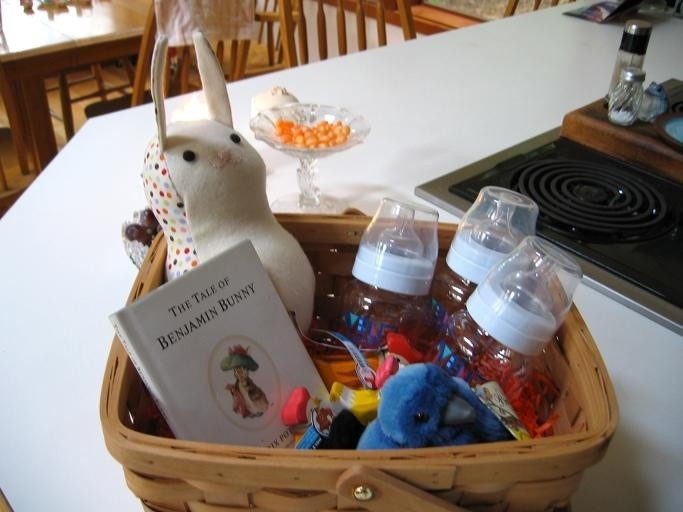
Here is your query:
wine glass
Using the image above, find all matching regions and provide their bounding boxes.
[251,103,368,220]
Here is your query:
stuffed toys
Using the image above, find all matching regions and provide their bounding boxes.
[140,26,319,339]
[280,329,428,451]
[354,360,522,451]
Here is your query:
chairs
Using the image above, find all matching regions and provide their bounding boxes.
[82,0,414,120]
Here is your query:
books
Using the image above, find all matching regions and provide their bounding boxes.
[105,236,337,448]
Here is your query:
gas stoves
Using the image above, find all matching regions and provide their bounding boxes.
[414,128,682,340]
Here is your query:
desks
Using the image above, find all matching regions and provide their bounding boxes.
[0,0,151,173]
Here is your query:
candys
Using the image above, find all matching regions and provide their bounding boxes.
[274,115,349,150]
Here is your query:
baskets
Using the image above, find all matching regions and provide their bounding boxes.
[97,212,621,512]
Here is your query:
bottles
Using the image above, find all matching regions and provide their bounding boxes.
[605,19,653,129]
[444,234,584,376]
[335,198,440,346]
[440,187,540,308]
